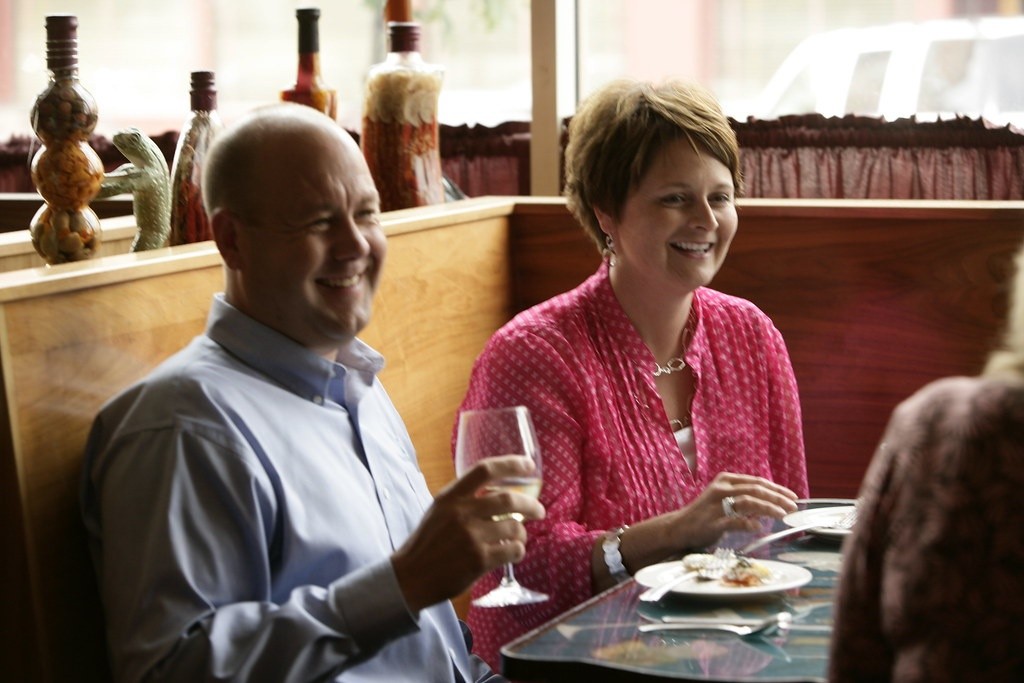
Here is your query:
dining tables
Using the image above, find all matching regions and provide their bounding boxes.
[497,497,859,683]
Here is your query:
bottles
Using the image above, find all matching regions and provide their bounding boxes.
[362,22,445,214]
[280,6,337,122]
[171,71,220,246]
[29,14,102,264]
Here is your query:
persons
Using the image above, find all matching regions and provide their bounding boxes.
[596,500,804,681]
[821,240,1024,683]
[84,102,548,683]
[451,78,810,673]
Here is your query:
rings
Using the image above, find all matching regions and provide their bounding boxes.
[721,496,739,520]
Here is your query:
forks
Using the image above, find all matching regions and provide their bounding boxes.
[638,547,743,603]
[734,509,862,555]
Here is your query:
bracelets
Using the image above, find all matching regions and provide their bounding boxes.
[601,525,629,583]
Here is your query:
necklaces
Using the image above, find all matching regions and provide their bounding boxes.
[650,327,697,429]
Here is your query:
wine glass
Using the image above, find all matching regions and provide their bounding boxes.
[454,405,553,609]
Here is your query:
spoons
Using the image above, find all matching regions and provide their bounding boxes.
[637,618,779,639]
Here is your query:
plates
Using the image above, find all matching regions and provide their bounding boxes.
[783,505,858,540]
[634,557,816,596]
